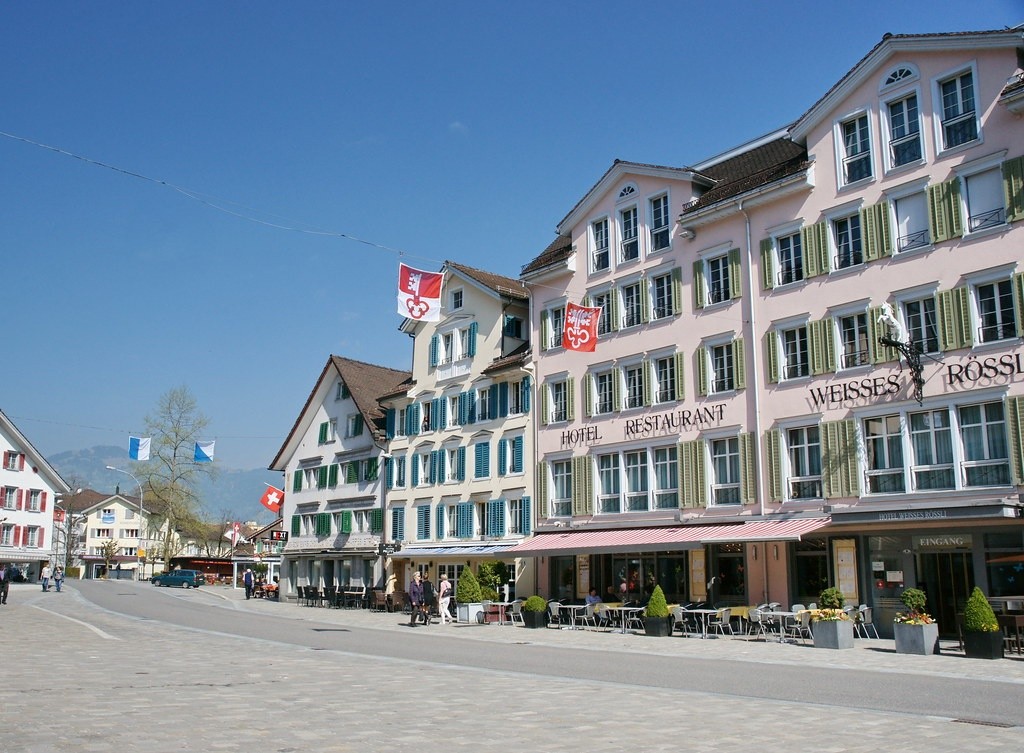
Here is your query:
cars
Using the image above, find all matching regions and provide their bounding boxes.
[4,568,22,579]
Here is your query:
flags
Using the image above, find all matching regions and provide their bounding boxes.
[128,436,152,460]
[561,301,602,352]
[194,439,215,462]
[397,262,444,322]
[260,485,285,513]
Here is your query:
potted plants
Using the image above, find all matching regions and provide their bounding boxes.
[894,588,940,655]
[520,596,550,629]
[455,557,511,624]
[640,584,675,637]
[812,587,854,649]
[960,586,1004,659]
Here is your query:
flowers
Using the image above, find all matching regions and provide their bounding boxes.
[894,611,936,625]
[812,609,849,623]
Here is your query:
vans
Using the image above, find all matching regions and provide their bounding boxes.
[151,570,205,588]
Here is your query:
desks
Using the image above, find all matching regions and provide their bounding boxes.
[594,603,624,627]
[260,585,277,598]
[955,612,1024,655]
[798,608,841,639]
[682,609,721,639]
[606,607,642,634]
[489,602,511,625]
[716,606,757,635]
[556,605,586,631]
[762,611,795,644]
[309,590,364,609]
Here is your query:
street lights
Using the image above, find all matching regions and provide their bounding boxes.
[106,466,142,582]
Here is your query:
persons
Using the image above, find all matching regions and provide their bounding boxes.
[386,575,398,613]
[422,572,434,626]
[437,574,453,624]
[40,562,52,592]
[586,587,607,623]
[54,565,65,592]
[242,569,255,600]
[409,571,425,627]
[0,563,10,605]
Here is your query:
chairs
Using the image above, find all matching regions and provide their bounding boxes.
[481,597,880,647]
[956,598,1024,650]
[253,584,279,599]
[297,586,412,614]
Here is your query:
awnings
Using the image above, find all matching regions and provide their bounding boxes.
[494,517,831,559]
[387,545,515,559]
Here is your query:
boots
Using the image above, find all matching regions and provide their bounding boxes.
[422,611,431,625]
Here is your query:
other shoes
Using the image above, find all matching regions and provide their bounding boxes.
[410,623,418,627]
[2,600,7,604]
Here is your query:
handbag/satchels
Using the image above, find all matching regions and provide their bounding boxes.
[441,588,452,598]
[432,588,439,597]
[418,610,424,621]
